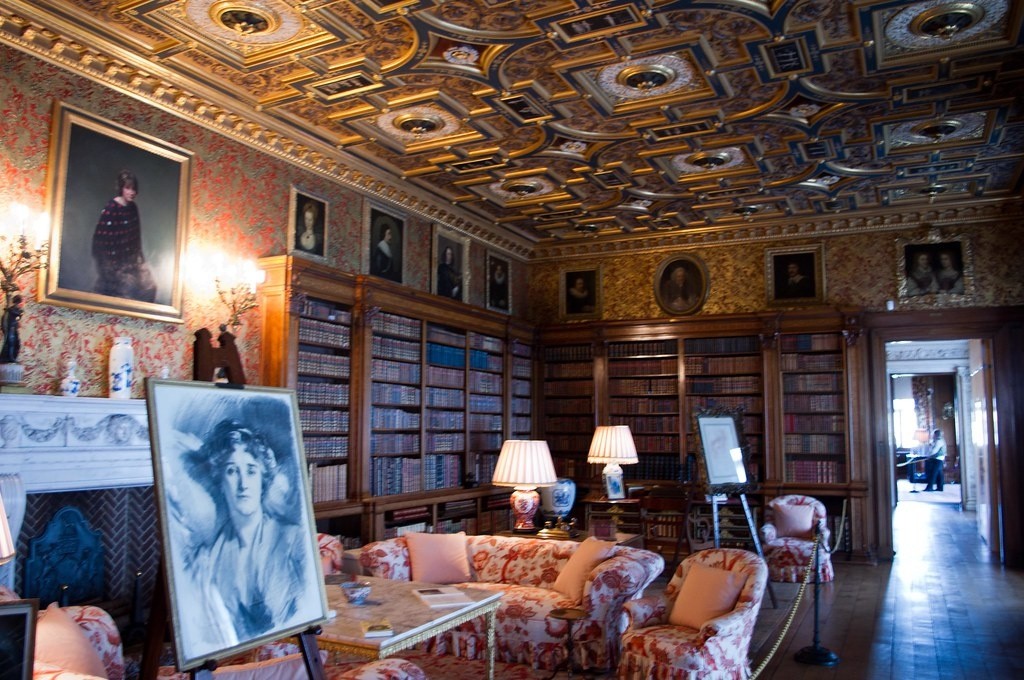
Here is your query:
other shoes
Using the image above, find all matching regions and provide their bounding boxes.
[934,488,943,491]
[923,488,933,491]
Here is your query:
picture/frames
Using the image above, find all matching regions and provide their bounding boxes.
[486,249,513,316]
[654,254,708,316]
[432,222,472,305]
[36,98,197,325]
[144,376,330,673]
[285,184,331,264]
[893,233,975,304]
[764,243,826,308]
[361,195,409,284]
[559,265,602,320]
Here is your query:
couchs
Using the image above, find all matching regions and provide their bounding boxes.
[615,547,769,680]
[360,531,665,670]
[758,495,834,582]
[61,605,425,680]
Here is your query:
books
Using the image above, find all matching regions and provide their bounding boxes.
[412,586,476,607]
[544,332,845,484]
[360,619,393,638]
[297,299,533,502]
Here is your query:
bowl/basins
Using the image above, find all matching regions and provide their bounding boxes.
[340,583,371,603]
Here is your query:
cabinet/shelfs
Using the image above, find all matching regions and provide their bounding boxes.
[257,255,881,567]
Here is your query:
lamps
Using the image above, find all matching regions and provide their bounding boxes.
[586,425,639,496]
[0,228,52,308]
[212,255,266,328]
[491,439,557,529]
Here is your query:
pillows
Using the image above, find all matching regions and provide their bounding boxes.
[32,600,328,680]
[552,535,616,605]
[405,530,471,583]
[773,501,814,538]
[666,562,748,631]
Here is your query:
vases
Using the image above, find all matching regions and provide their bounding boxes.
[534,477,577,526]
[59,359,81,397]
[108,337,135,399]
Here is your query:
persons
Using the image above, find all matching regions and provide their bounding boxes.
[923,429,947,491]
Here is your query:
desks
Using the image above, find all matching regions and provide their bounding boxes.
[271,569,505,680]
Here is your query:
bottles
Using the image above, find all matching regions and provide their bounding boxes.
[108,337,134,399]
[60,362,81,397]
[538,479,576,521]
